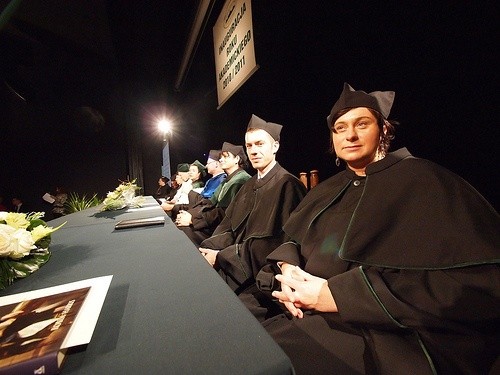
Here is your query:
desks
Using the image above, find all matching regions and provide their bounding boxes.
[0,196,296,375]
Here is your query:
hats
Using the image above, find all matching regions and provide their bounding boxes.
[222,142,247,163]
[326,87,382,129]
[179,164,191,172]
[245,114,283,141]
[209,150,222,161]
[194,160,206,172]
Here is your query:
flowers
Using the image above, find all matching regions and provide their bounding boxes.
[102,178,145,212]
[0,211,67,290]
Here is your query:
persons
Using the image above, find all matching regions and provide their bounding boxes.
[239,82,500,375]
[155,142,251,246]
[200,113,307,323]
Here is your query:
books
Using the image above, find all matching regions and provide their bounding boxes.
[0,347,69,375]
[115,217,164,230]
